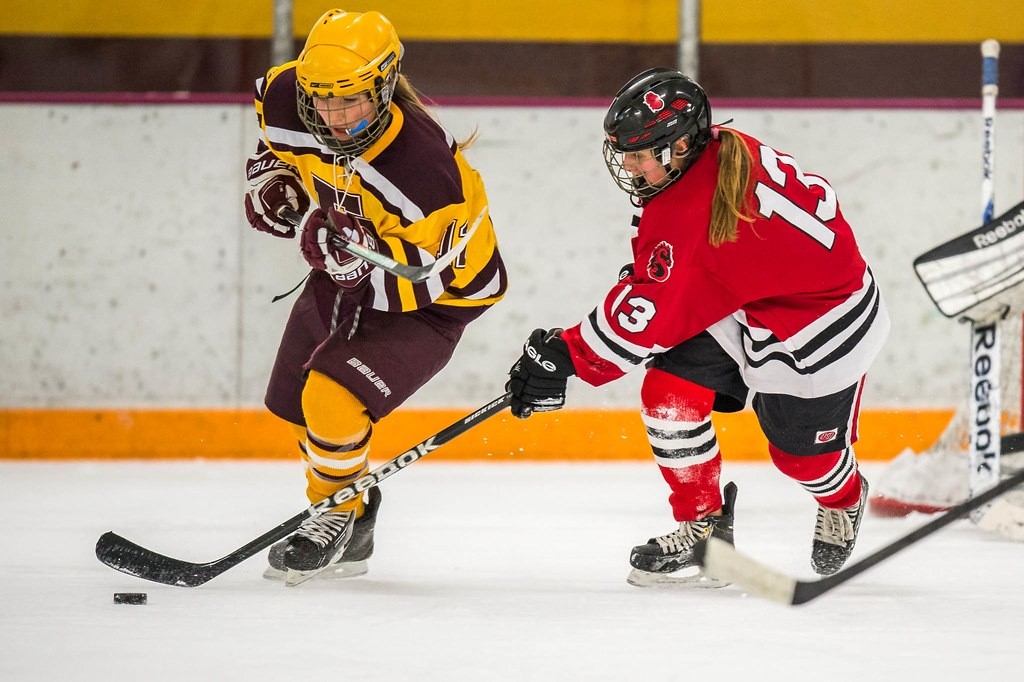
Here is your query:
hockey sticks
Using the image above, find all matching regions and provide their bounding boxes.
[965,37,1022,537]
[691,466,1024,606]
[276,205,491,283]
[94,391,511,586]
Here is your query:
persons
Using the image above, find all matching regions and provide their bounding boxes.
[244,8,509,588]
[504,67,892,590]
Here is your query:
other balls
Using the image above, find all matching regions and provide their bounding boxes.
[113,592,148,606]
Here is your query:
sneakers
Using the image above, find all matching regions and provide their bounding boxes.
[811,474,868,575]
[281,508,355,587]
[263,485,382,581]
[626,482,739,588]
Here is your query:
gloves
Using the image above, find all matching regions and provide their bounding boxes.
[301,207,380,289]
[244,149,310,238]
[505,328,577,419]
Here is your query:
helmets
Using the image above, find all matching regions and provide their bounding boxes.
[602,68,712,199]
[296,8,404,157]
